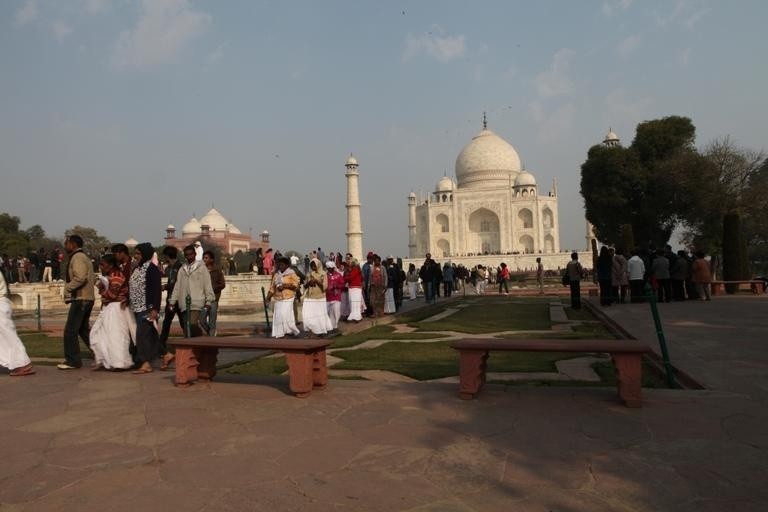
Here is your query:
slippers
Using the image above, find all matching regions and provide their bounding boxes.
[132,369,152,373]
[159,354,175,370]
[9,366,35,375]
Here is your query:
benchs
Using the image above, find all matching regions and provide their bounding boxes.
[711,280,764,296]
[450,336,651,409]
[165,335,334,398]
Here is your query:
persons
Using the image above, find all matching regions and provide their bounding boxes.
[646,244,661,295]
[111,244,137,275]
[406,264,418,302]
[168,246,215,338]
[57,234,95,369]
[612,248,628,303]
[263,249,273,275]
[596,246,612,308]
[627,250,645,304]
[362,252,374,317]
[0,256,36,375]
[673,250,688,301]
[304,247,353,267]
[497,243,591,259]
[51,246,64,283]
[89,254,138,370]
[227,250,242,275]
[160,246,185,341]
[499,263,509,293]
[692,252,712,300]
[325,261,344,327]
[42,254,52,282]
[267,258,300,338]
[649,250,673,303]
[302,258,333,335]
[420,250,497,304]
[369,256,388,318]
[344,259,362,322]
[274,250,283,265]
[536,257,545,294]
[0,249,39,283]
[665,245,676,287]
[199,251,226,336]
[120,242,176,374]
[255,247,264,275]
[566,253,583,310]
[286,257,306,325]
[382,254,408,314]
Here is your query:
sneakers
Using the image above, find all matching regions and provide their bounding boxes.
[57,362,76,369]
